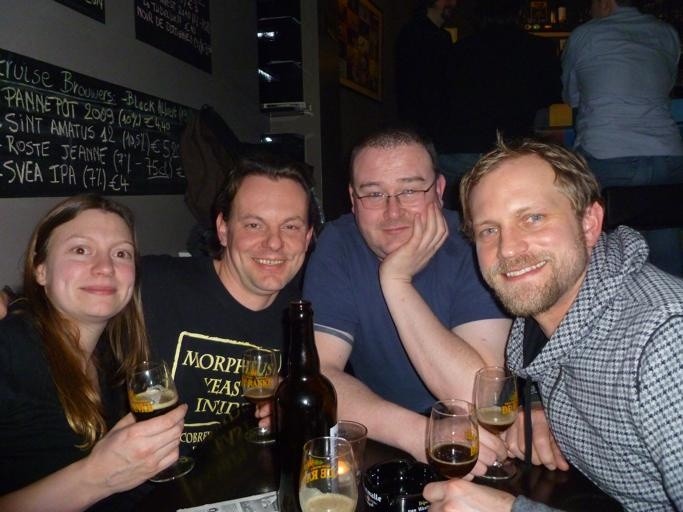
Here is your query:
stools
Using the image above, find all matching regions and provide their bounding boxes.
[600,185,683,231]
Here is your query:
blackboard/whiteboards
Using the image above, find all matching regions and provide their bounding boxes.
[1,46,199,198]
[131,1,213,71]
[62,1,105,22]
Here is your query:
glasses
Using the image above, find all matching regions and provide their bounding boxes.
[350,176,437,208]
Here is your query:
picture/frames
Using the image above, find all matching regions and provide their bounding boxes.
[336,0,384,103]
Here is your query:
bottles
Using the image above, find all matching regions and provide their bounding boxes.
[273,301,337,512]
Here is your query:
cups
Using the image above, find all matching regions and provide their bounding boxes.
[427,398,480,482]
[299,437,362,512]
[335,421,367,487]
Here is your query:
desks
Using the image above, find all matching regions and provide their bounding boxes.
[131,405,629,512]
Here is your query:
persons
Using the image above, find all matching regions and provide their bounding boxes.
[401,0,562,177]
[301,133,514,482]
[1,193,188,512]
[424,139,683,510]
[0,159,319,477]
[560,2,682,186]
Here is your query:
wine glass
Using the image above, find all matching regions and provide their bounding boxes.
[240,350,279,447]
[474,367,519,480]
[125,360,194,485]
